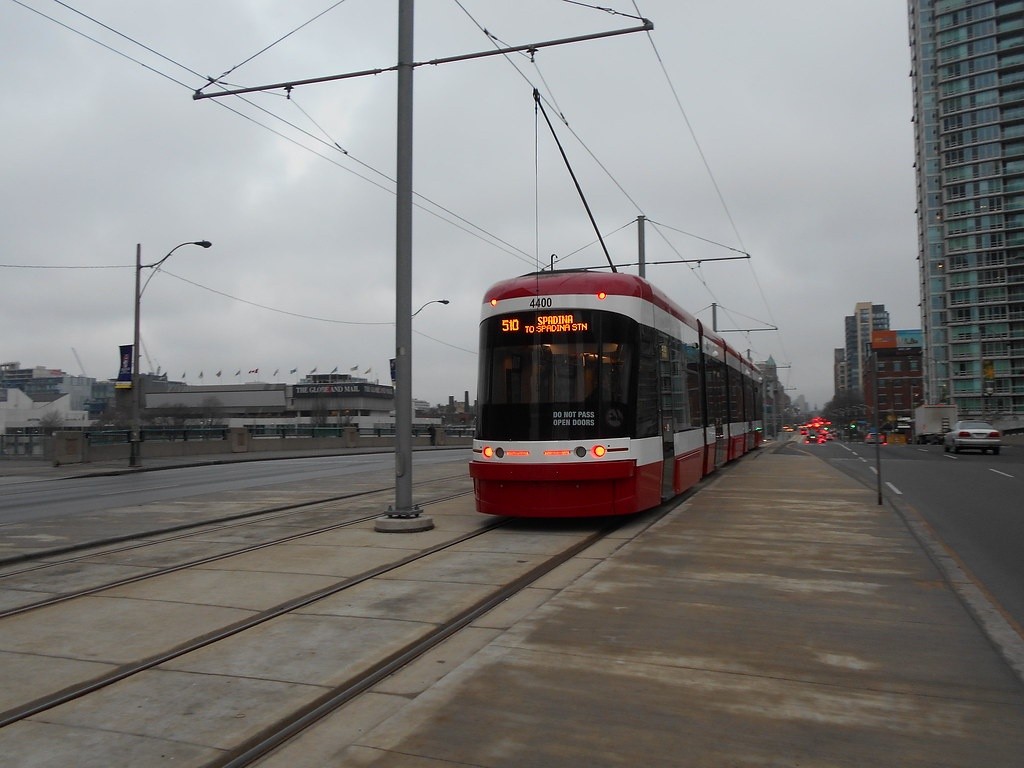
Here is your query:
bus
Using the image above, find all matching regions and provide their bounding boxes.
[465,267,765,518]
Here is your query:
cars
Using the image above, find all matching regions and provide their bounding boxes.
[942,419,1002,455]
[865,433,885,444]
[881,417,915,433]
[783,423,863,445]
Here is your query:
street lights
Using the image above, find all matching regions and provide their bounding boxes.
[941,320,1014,416]
[128,239,213,467]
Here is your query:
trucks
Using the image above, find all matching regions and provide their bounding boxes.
[908,404,958,445]
